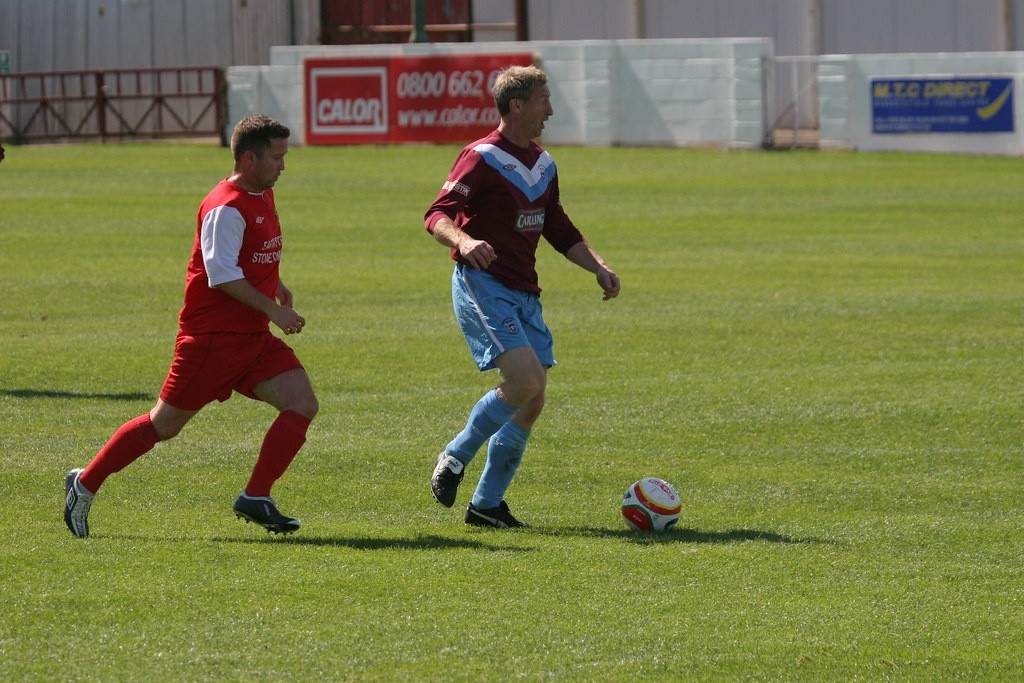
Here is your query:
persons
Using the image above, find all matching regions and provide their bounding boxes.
[63,113,319,541]
[424,64,621,529]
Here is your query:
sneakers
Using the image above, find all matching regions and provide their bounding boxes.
[233,487,301,535]
[430,449,465,509]
[464,499,532,531]
[63,467,95,538]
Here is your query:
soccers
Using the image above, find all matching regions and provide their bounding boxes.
[621,476,683,533]
